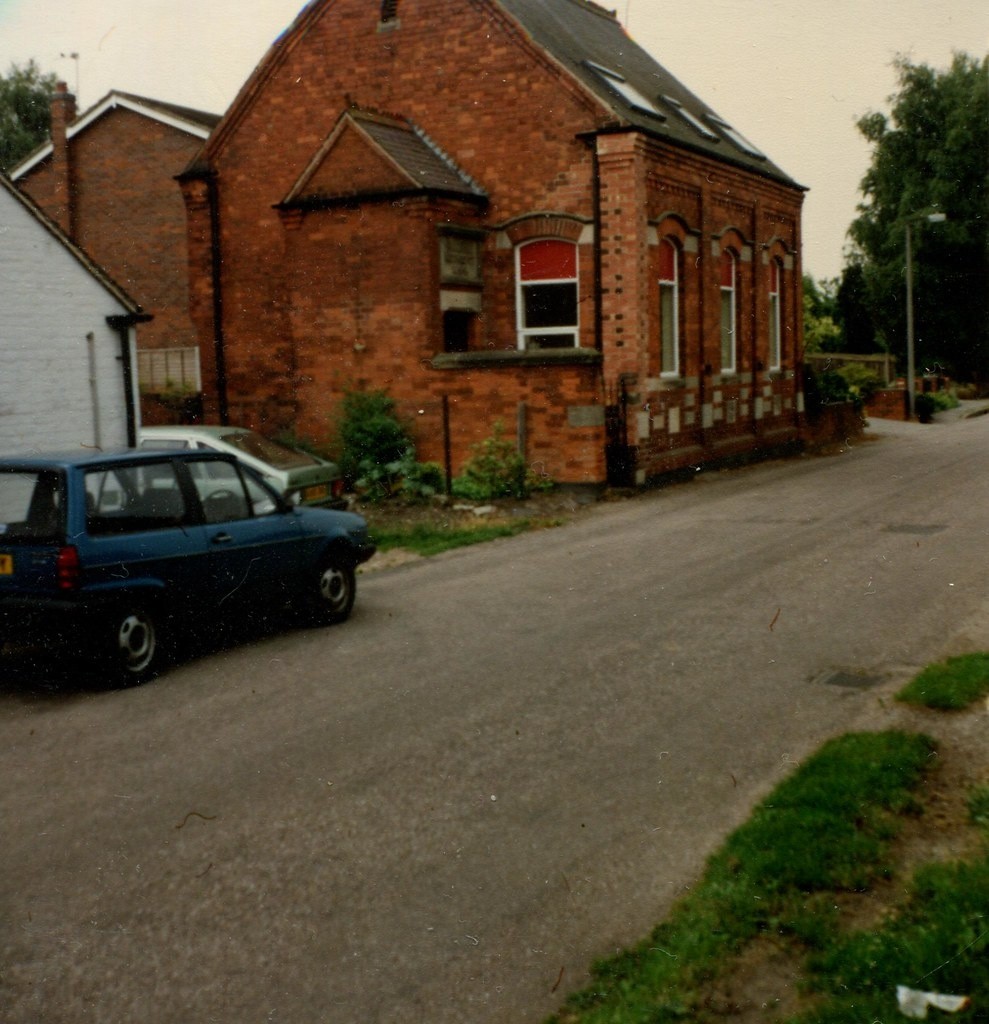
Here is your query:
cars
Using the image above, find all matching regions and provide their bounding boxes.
[0,445,372,690]
[138,425,350,518]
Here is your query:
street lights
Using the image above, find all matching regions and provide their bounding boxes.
[904,213,946,421]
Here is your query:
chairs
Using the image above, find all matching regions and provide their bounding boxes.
[140,487,178,517]
